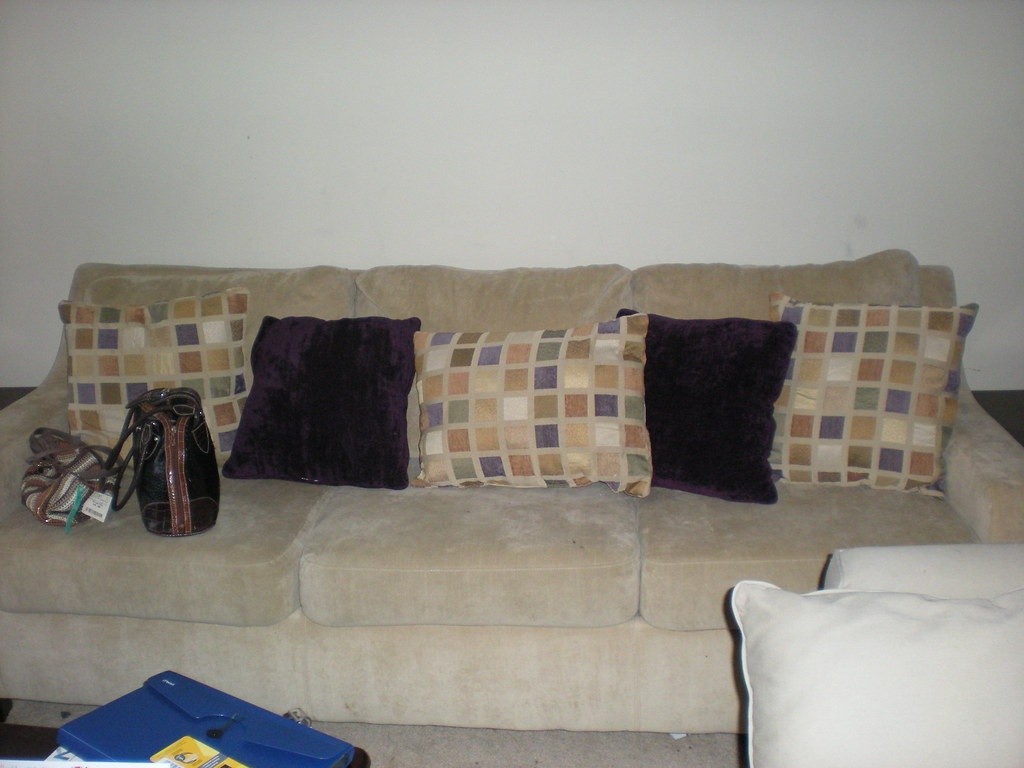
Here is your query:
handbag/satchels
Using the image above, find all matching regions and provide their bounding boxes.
[106,387,223,537]
[19,426,113,524]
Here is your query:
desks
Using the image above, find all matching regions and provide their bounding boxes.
[0,724,370,768]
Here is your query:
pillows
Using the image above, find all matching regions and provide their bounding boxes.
[55,287,255,471]
[770,298,980,497]
[722,582,1024,768]
[613,310,796,504]
[222,317,421,492]
[413,313,654,497]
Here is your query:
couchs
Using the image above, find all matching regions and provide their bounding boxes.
[0,247,1024,733]
[827,544,1024,603]
